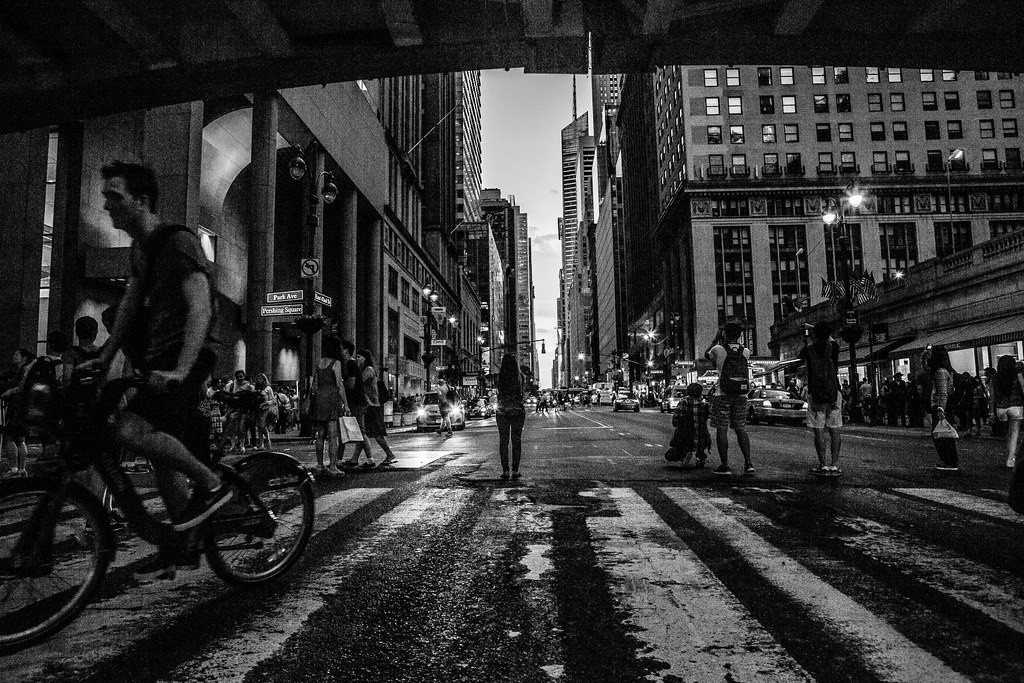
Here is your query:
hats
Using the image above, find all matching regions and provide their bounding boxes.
[893,372,904,377]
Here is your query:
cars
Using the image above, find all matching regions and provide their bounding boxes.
[414,389,537,433]
[573,382,641,412]
[745,387,809,428]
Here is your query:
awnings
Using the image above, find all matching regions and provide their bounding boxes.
[888,311,1024,360]
[753,358,801,379]
[796,340,898,374]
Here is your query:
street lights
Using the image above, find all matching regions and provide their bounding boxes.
[421,283,438,391]
[452,317,459,386]
[476,337,486,397]
[817,179,868,425]
[944,150,964,254]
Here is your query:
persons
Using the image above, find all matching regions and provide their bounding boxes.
[840,362,1024,438]
[536,389,575,414]
[612,387,617,407]
[1,305,134,549]
[496,353,526,478]
[777,380,808,402]
[920,345,958,470]
[704,322,756,475]
[672,383,709,469]
[436,379,453,436]
[596,391,601,407]
[793,321,844,477]
[308,333,398,478]
[198,371,301,453]
[990,355,1024,467]
[74,163,234,581]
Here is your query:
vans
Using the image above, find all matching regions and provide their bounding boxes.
[660,385,687,415]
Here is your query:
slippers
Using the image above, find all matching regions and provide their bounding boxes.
[317,455,398,476]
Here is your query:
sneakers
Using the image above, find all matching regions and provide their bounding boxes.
[173,478,233,533]
[121,551,200,583]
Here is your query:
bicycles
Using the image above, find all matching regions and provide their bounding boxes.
[0,355,318,656]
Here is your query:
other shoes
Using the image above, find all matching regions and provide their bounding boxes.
[435,429,453,437]
[934,463,958,471]
[679,453,755,475]
[512,473,522,477]
[830,465,842,475]
[1006,458,1016,467]
[500,474,510,479]
[812,465,828,474]
[3,469,28,478]
[227,439,271,452]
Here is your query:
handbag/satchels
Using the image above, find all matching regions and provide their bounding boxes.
[339,412,364,444]
[932,407,959,439]
[377,380,391,403]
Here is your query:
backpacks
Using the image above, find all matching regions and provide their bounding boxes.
[24,356,63,415]
[807,343,838,403]
[719,343,749,395]
[446,387,460,406]
[948,371,970,409]
